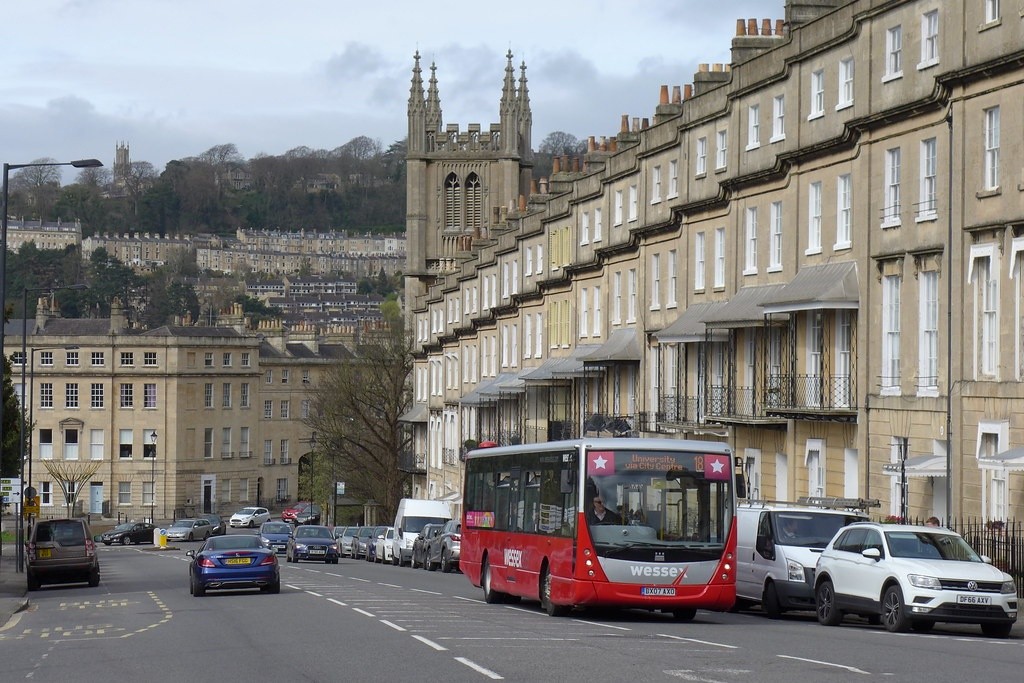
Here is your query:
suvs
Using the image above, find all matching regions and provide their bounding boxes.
[22,517,102,590]
[425,519,466,573]
[411,523,447,569]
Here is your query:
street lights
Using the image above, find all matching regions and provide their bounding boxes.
[20,284,92,572]
[28,344,79,540]
[150,431,159,523]
[0,159,104,369]
[308,436,317,525]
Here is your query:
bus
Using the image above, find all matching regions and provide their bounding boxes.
[459,439,737,622]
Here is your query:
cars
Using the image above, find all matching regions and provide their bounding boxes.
[813,522,1018,638]
[286,524,344,564]
[186,535,283,598]
[230,507,272,528]
[282,501,316,523]
[258,521,294,552]
[333,527,347,544]
[377,528,394,564]
[366,526,391,563]
[166,518,212,542]
[340,526,360,559]
[351,526,377,560]
[294,505,322,528]
[203,516,228,534]
[101,522,161,545]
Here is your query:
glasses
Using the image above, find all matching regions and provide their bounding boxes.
[595,501,607,505]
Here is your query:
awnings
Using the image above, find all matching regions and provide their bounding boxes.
[518,358,571,386]
[882,455,947,477]
[495,367,538,392]
[757,262,859,311]
[397,403,427,422]
[978,447,1024,471]
[653,302,730,342]
[576,327,642,362]
[459,374,520,407]
[551,344,606,378]
[698,284,789,328]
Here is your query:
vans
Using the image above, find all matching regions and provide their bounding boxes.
[392,498,454,568]
[733,501,875,620]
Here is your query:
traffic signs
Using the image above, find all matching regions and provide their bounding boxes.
[0,479,22,504]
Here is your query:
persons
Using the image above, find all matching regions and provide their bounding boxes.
[926,517,939,527]
[588,495,624,525]
[782,519,799,538]
